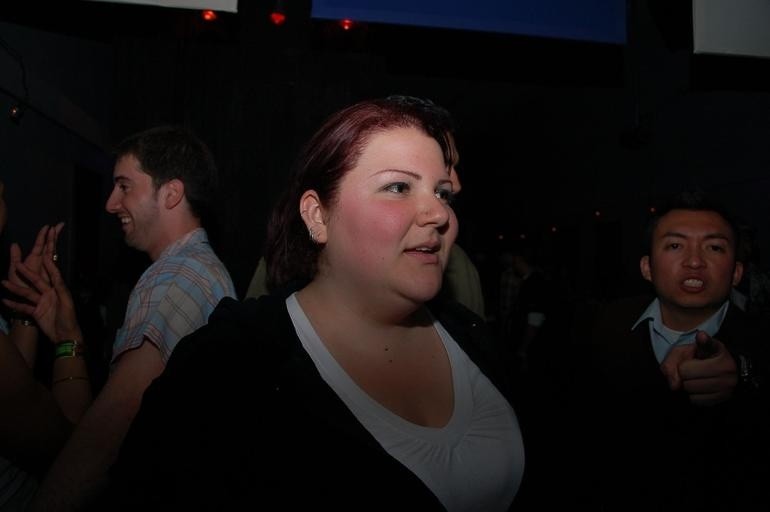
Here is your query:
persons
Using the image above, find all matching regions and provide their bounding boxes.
[1,90,768,511]
